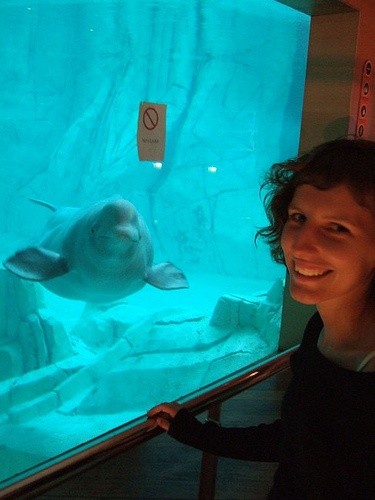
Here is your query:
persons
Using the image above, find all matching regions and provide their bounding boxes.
[145,132,375,500]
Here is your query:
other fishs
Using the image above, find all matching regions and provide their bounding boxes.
[2,198,188,302]
